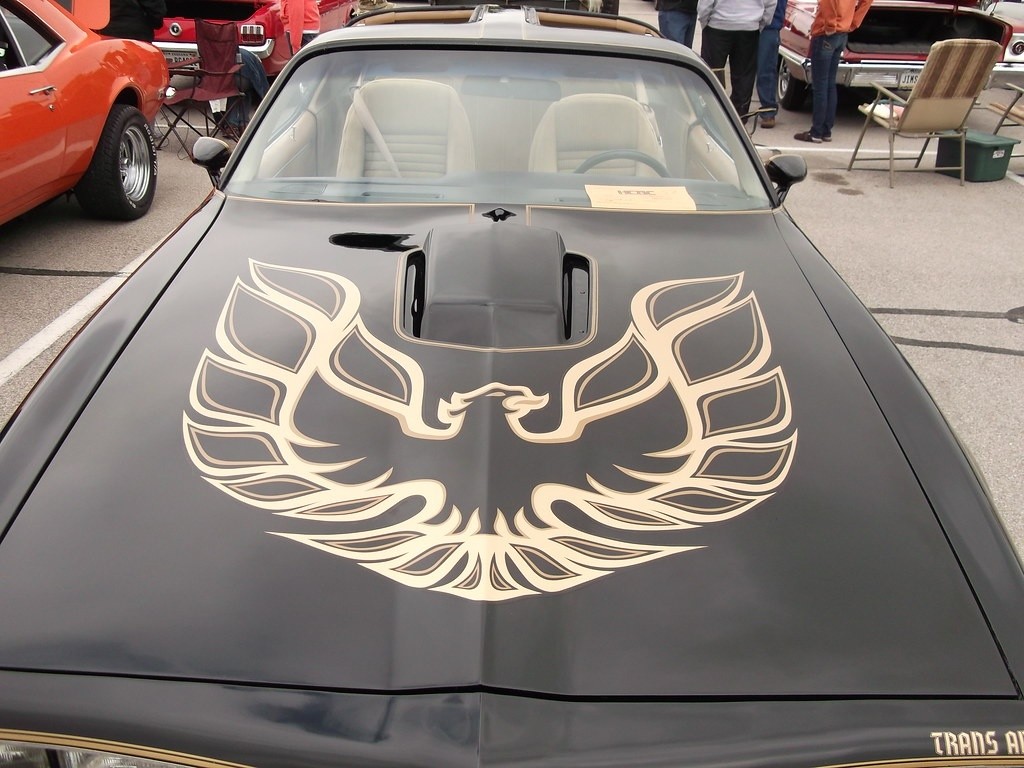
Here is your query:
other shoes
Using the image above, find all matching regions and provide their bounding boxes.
[223,124,236,137]
[239,123,247,136]
[794,131,822,143]
[822,136,832,142]
[761,116,775,128]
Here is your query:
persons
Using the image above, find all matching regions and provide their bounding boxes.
[795,0,872,143]
[656,0,698,50]
[697,0,778,126]
[757,0,788,127]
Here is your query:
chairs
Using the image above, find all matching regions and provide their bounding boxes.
[340,79,475,182]
[530,92,665,177]
[846,37,1004,189]
[151,19,249,161]
[986,83,1024,157]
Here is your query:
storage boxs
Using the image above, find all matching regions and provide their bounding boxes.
[934,130,1021,183]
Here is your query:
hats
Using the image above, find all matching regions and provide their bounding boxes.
[351,0,396,16]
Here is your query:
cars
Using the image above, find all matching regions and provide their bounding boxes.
[0,0,1024,768]
[776,0,1024,112]
[0,0,179,227]
[152,0,359,79]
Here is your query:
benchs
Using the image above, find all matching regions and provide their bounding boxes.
[348,69,645,176]
